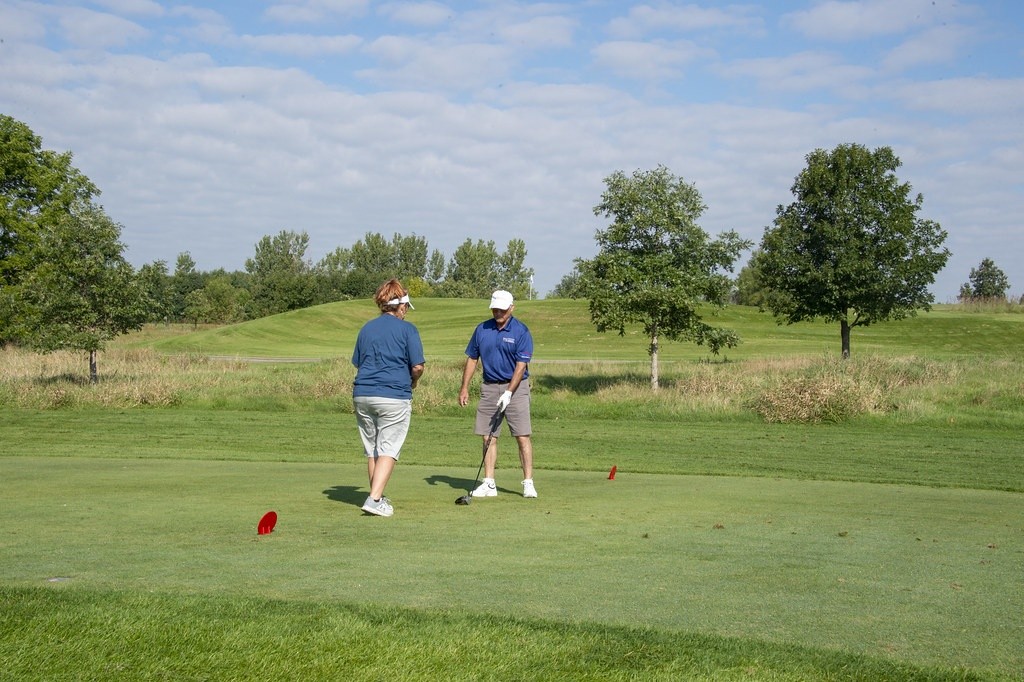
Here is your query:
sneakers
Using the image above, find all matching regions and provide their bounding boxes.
[469,478,497,497]
[360,496,394,517]
[520,478,537,498]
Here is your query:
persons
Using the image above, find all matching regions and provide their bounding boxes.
[351,279,426,517]
[458,290,537,498]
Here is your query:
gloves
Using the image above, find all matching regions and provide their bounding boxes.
[496,390,512,413]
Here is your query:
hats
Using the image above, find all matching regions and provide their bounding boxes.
[382,294,416,311]
[488,290,513,310]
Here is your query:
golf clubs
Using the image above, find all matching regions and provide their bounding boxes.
[453,407,504,505]
